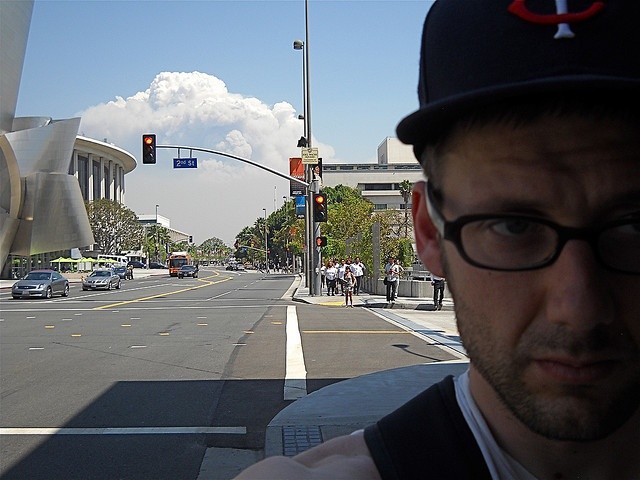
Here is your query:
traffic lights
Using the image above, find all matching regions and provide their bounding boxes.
[142,134,156,165]
[316,237,327,246]
[313,193,327,222]
[189,236,192,242]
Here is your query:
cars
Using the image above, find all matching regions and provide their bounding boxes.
[178,265,199,279]
[82,270,121,290]
[12,270,69,300]
[114,267,132,280]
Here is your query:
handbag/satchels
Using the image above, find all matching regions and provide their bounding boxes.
[383,264,395,284]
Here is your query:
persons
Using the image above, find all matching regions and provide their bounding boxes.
[320,257,367,309]
[430,271,445,309]
[384,256,400,305]
[312,165,321,180]
[126,263,134,280]
[230,1,640,480]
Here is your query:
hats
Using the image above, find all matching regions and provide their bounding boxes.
[396,0,639,145]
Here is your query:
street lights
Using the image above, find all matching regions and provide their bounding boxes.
[263,209,267,272]
[283,196,288,221]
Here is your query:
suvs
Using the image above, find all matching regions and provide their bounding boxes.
[127,261,144,267]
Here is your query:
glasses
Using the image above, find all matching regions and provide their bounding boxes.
[425,183,639,271]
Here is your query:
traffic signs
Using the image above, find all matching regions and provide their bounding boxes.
[173,158,197,169]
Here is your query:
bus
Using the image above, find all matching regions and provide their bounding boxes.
[98,256,128,268]
[170,252,190,276]
